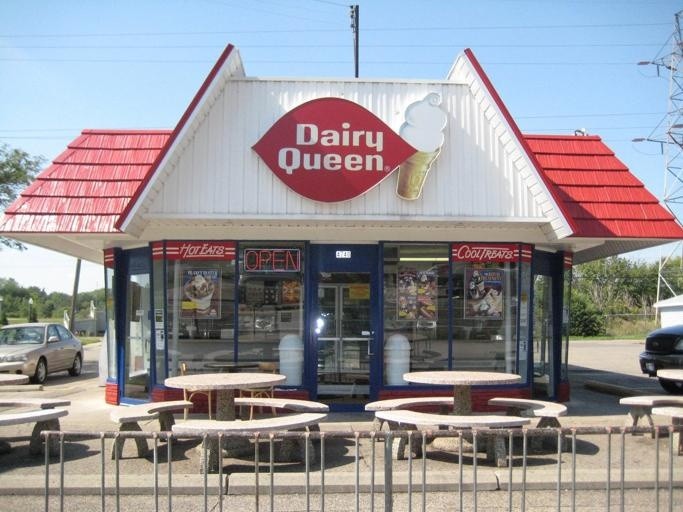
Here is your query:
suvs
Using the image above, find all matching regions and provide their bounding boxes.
[639,323,683,396]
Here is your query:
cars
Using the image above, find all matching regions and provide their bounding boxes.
[0,322,84,384]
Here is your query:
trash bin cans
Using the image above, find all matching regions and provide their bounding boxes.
[278,333,304,386]
[383,333,412,385]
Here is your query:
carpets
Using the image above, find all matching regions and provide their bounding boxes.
[326,403,365,412]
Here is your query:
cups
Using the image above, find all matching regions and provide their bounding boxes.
[396,149,441,201]
[183,279,215,314]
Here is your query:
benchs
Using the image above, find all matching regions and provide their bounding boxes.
[0,398,71,442]
[111,400,194,461]
[0,409,66,457]
[173,412,327,474]
[377,410,531,469]
[619,395,683,438]
[651,406,683,455]
[488,396,569,449]
[233,397,330,450]
[364,396,454,442]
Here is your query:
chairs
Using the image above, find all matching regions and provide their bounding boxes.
[180,361,213,420]
[237,363,277,419]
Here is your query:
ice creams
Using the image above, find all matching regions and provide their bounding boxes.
[396,91,447,200]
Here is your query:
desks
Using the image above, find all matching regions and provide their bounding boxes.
[404,370,521,452]
[163,373,288,458]
[656,369,683,380]
[205,362,259,417]
